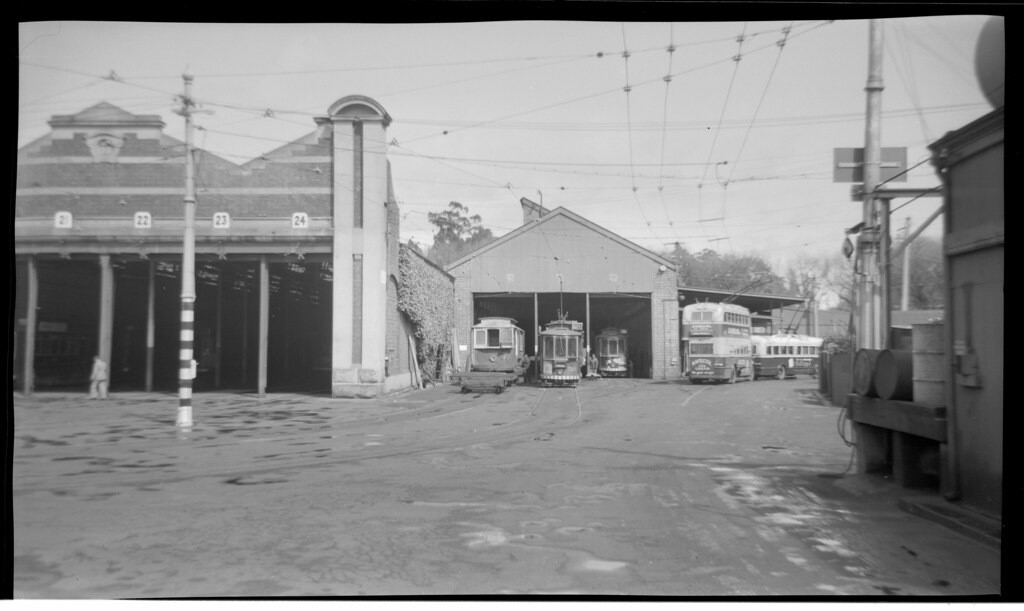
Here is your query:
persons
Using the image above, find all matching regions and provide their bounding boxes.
[590,353,599,374]
[86,356,108,400]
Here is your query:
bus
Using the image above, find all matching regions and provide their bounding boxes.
[459,315,524,384]
[679,278,772,384]
[750,291,825,382]
[538,307,586,388]
[595,326,634,379]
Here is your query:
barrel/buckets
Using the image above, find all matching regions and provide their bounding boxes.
[853,323,946,406]
